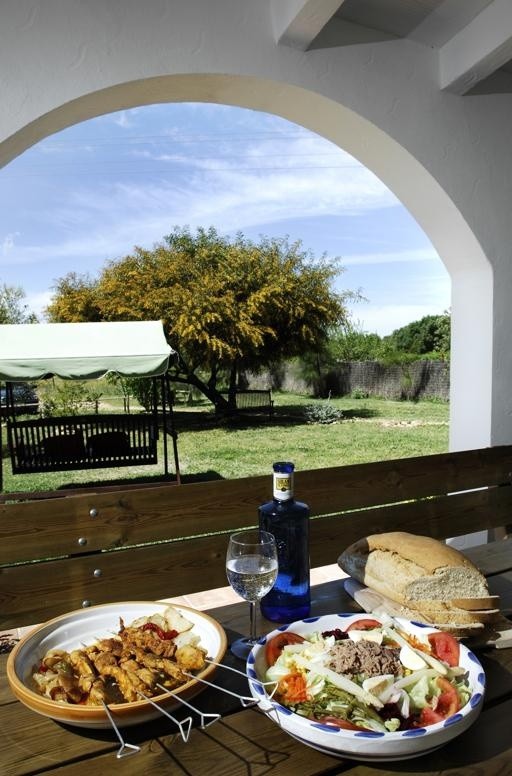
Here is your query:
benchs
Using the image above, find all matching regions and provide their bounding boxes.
[5,380,39,407]
[215,388,274,418]
[8,411,158,475]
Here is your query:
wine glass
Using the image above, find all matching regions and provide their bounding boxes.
[224,529,278,661]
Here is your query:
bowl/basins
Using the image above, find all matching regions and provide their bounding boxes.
[244,611,487,764]
[5,598,230,732]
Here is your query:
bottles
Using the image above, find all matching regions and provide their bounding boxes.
[258,460,312,624]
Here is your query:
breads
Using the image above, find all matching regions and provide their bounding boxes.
[337,530,501,635]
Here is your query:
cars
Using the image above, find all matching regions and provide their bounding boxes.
[1,380,41,414]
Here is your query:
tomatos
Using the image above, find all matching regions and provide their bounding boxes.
[344,619,380,635]
[427,630,461,667]
[265,630,306,665]
[411,675,462,727]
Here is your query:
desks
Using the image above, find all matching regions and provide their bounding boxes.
[0,444,512,776]
